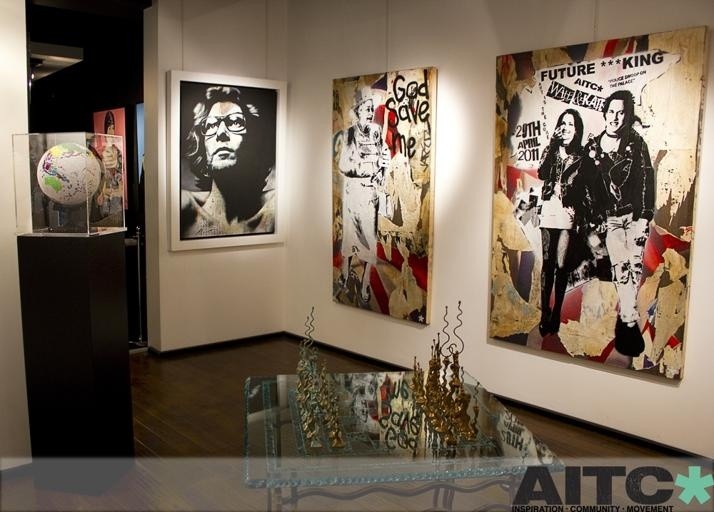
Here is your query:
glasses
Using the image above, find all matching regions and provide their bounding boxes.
[200,111,247,135]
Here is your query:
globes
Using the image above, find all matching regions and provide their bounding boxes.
[36,143,102,232]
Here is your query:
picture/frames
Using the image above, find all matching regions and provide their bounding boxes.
[166,69,288,253]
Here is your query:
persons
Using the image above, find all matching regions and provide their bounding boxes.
[184,87,276,238]
[338,86,392,302]
[97,112,123,214]
[585,88,654,358]
[536,109,587,337]
